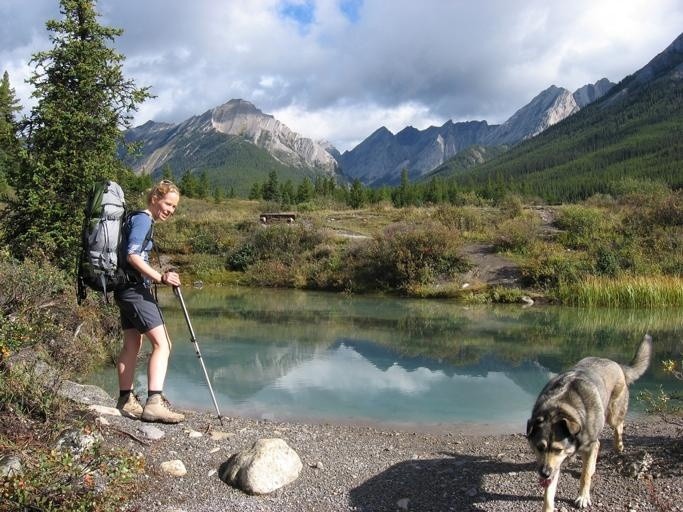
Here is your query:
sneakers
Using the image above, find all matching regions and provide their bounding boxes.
[140,391,185,424]
[115,391,143,419]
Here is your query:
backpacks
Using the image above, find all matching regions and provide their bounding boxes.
[73,177,158,307]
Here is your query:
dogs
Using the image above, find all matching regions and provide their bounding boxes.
[527,333,653,512]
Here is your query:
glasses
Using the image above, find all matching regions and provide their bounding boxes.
[153,178,173,196]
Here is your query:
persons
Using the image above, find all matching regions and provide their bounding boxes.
[116,179,185,424]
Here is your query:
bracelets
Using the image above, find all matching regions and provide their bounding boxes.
[161,272,166,283]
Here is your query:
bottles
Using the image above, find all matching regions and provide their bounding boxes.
[143,277,153,289]
